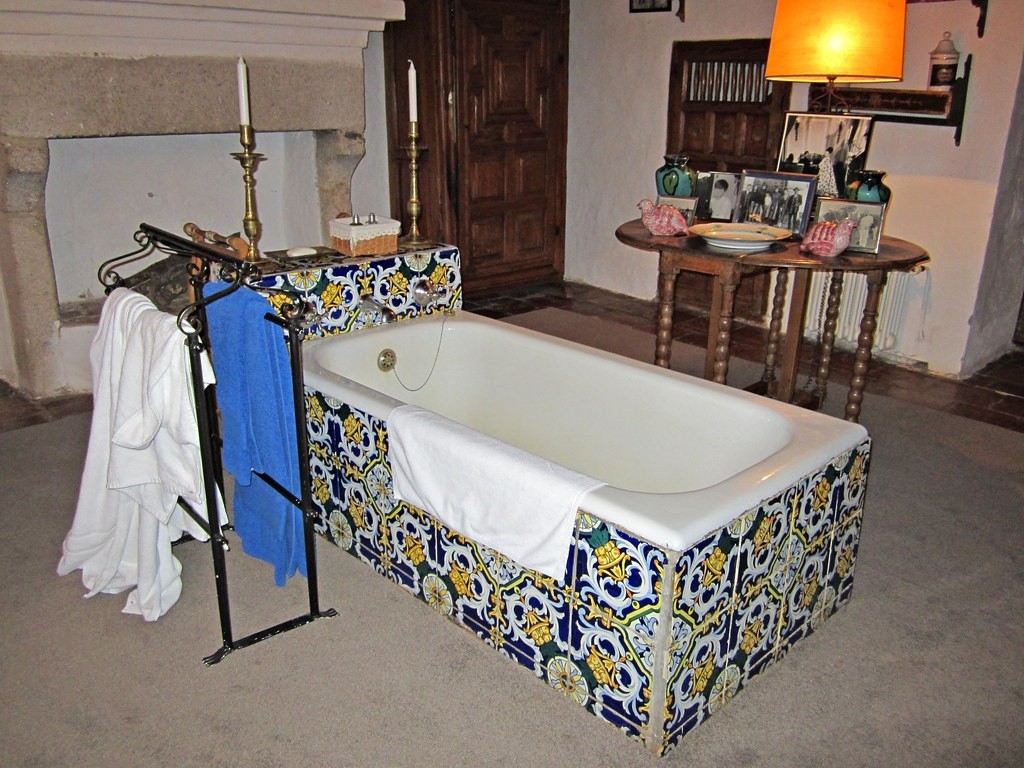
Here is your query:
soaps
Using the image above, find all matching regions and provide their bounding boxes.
[288,245,317,259]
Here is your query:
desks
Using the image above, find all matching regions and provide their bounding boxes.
[614,218,927,425]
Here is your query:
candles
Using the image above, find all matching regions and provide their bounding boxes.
[237,54,250,126]
[408,59,418,122]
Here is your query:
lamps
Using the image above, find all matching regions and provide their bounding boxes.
[764,1,907,112]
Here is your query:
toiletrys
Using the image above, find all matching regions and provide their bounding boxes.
[350,212,379,230]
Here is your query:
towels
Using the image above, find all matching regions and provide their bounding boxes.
[54,285,228,621]
[385,403,611,580]
[203,279,317,583]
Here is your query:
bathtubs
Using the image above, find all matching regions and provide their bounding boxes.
[296,311,873,754]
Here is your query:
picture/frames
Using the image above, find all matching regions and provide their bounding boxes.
[655,194,699,235]
[702,171,741,221]
[810,197,886,255]
[732,169,819,239]
[776,111,876,198]
[629,0,672,13]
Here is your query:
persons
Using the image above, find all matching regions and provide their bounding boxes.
[798,147,841,199]
[738,182,802,231]
[823,206,874,248]
[707,178,732,220]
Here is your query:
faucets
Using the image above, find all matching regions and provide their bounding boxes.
[358,295,398,325]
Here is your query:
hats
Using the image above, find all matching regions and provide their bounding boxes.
[793,186,800,192]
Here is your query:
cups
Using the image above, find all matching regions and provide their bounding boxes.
[927,55,960,92]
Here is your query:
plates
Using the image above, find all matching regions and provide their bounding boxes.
[689,222,792,249]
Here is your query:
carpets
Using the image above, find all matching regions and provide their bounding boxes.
[0,307,1024,768]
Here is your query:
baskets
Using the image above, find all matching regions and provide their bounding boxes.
[328,213,402,257]
